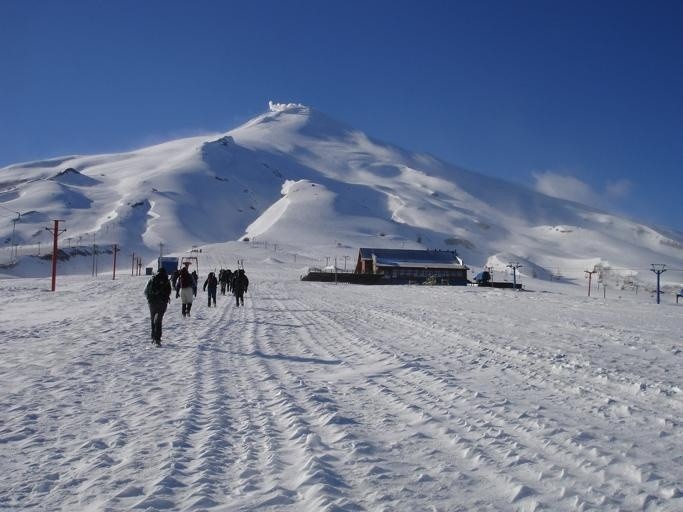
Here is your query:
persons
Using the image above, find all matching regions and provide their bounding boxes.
[170,268,249,317]
[143,268,172,347]
